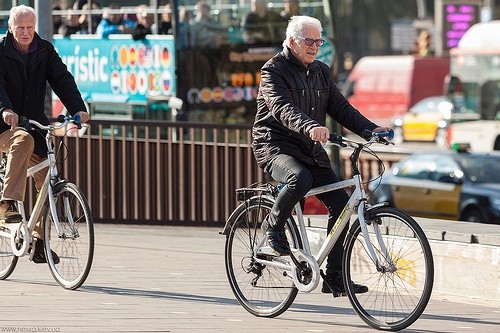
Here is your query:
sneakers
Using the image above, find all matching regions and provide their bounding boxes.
[0,200,22,220]
[322,279,369,294]
[260,214,292,254]
[28,241,60,264]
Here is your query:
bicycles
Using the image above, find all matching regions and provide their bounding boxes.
[222,129,434,330]
[0,116,94,293]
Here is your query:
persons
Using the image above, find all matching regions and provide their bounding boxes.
[0,5,90,264]
[51,0,432,97]
[251,15,393,293]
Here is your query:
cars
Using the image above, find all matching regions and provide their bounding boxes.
[370,153,500,223]
[392,96,452,142]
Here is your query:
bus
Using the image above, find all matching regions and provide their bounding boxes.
[442,21,500,153]
[0,1,335,120]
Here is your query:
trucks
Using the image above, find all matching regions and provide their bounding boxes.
[342,56,450,128]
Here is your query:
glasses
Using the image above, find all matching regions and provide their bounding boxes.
[298,35,325,47]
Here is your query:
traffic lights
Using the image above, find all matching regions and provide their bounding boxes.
[414,26,434,56]
